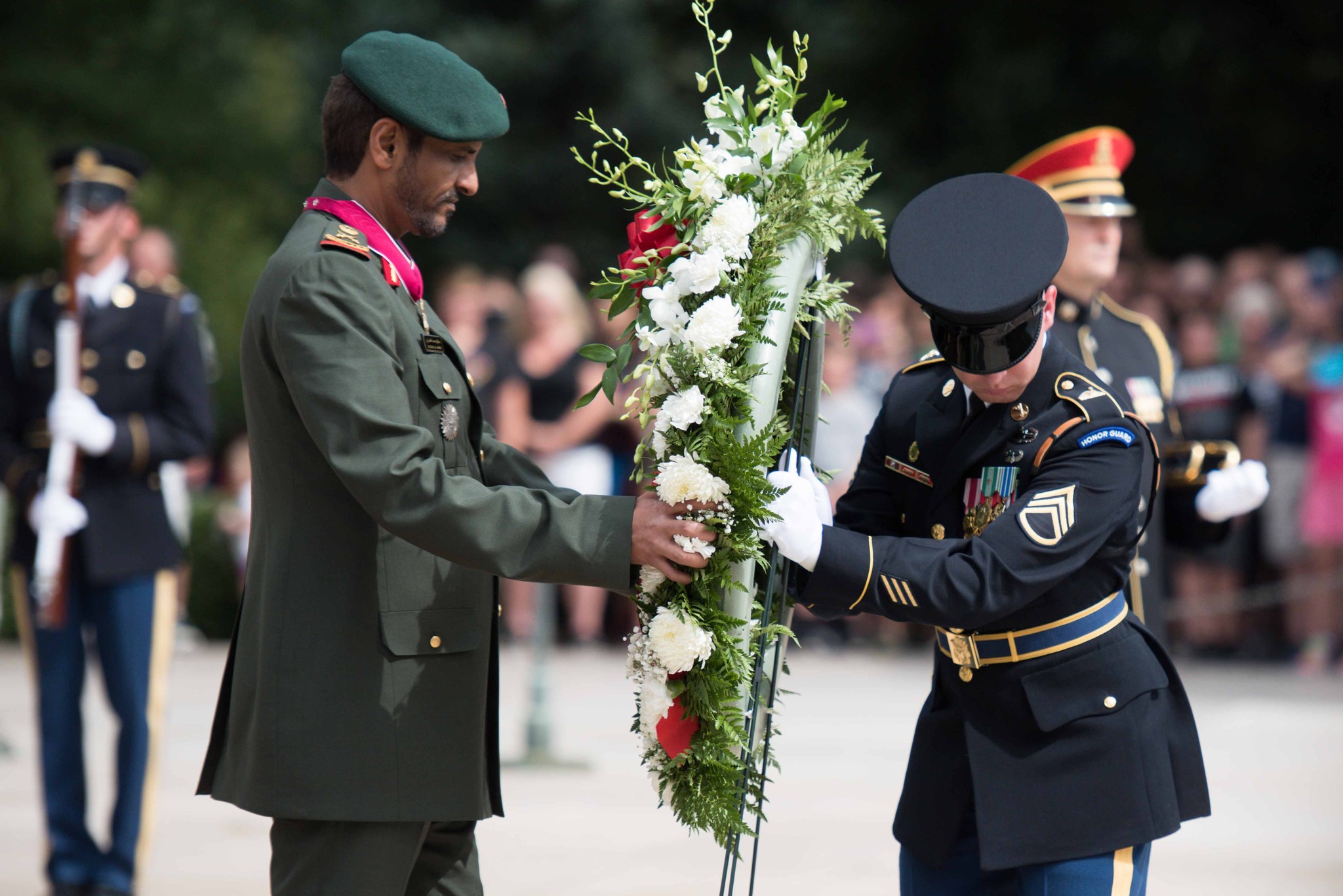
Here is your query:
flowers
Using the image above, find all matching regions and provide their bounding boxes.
[571,0,887,859]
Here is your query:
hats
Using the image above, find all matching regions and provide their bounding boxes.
[46,138,147,209]
[1003,126,1137,217]
[890,172,1069,374]
[339,31,510,142]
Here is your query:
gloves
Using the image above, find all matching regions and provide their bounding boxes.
[1192,457,1269,523]
[27,489,90,536]
[796,456,835,527]
[762,444,825,572]
[45,388,116,458]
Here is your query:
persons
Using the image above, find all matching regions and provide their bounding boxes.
[917,124,1270,647]
[125,225,1342,676]
[1,145,215,896]
[757,173,1212,896]
[196,30,717,896]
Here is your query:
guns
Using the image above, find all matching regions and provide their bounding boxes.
[30,160,80,629]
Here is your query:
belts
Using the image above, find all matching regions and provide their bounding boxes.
[933,590,1130,667]
